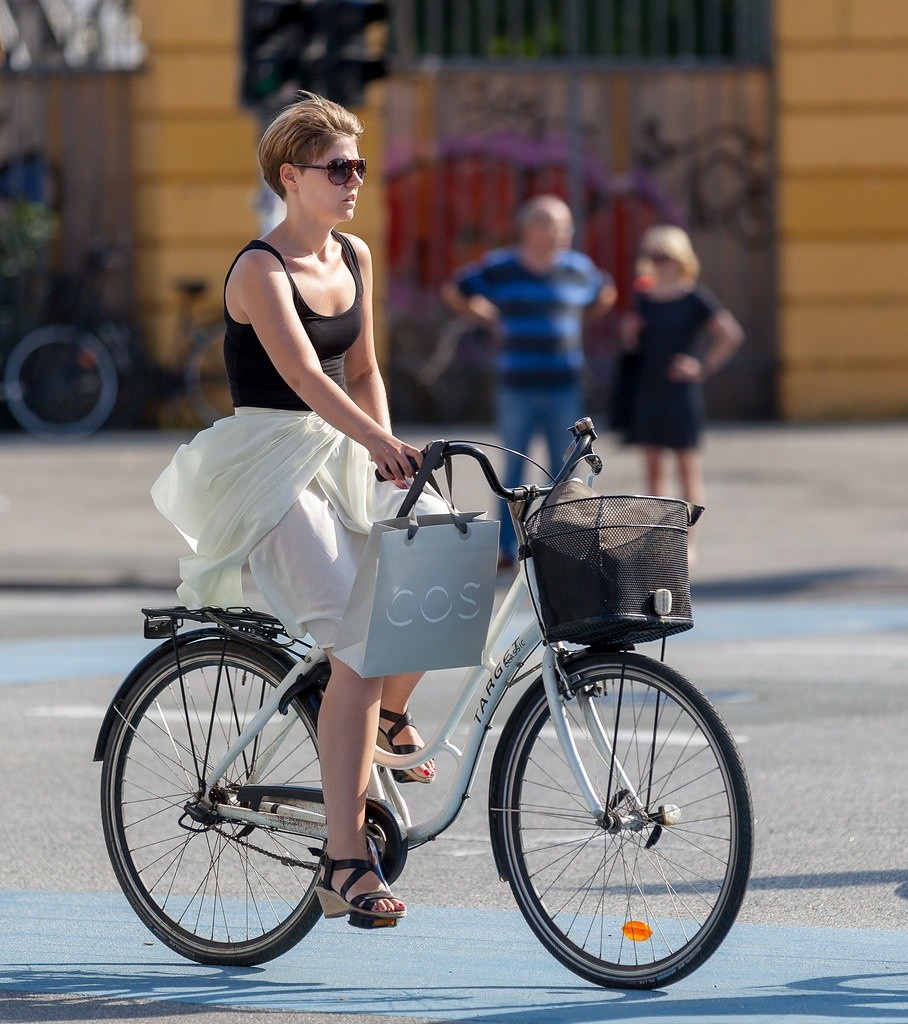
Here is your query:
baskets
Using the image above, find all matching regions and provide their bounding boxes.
[528,495,694,646]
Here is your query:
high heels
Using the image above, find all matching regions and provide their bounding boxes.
[375,707,435,784]
[313,853,407,919]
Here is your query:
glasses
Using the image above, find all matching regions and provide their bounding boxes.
[645,252,674,266]
[291,158,368,187]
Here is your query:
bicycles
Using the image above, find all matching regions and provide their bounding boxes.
[387,289,497,415]
[6,245,234,443]
[94,415,752,990]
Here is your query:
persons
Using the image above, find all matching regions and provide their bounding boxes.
[0,109,64,361]
[609,224,746,567]
[147,88,457,921]
[389,194,618,571]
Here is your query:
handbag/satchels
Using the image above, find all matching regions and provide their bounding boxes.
[330,441,500,678]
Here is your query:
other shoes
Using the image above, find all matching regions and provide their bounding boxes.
[496,554,514,572]
